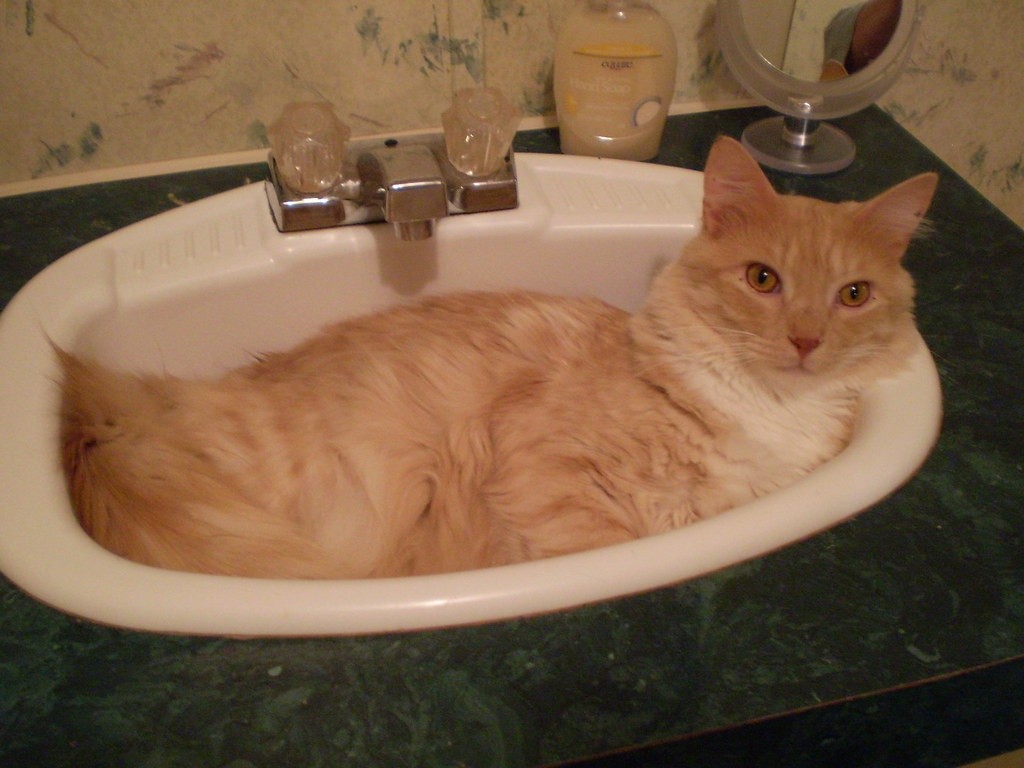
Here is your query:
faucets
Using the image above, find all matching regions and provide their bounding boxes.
[355,136,452,242]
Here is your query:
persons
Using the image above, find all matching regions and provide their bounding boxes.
[820,0,902,82]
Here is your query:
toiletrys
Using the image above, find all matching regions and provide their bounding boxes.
[551,1,679,164]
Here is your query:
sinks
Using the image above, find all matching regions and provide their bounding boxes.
[0,148,943,640]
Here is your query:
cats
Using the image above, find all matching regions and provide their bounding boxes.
[44,134,939,580]
